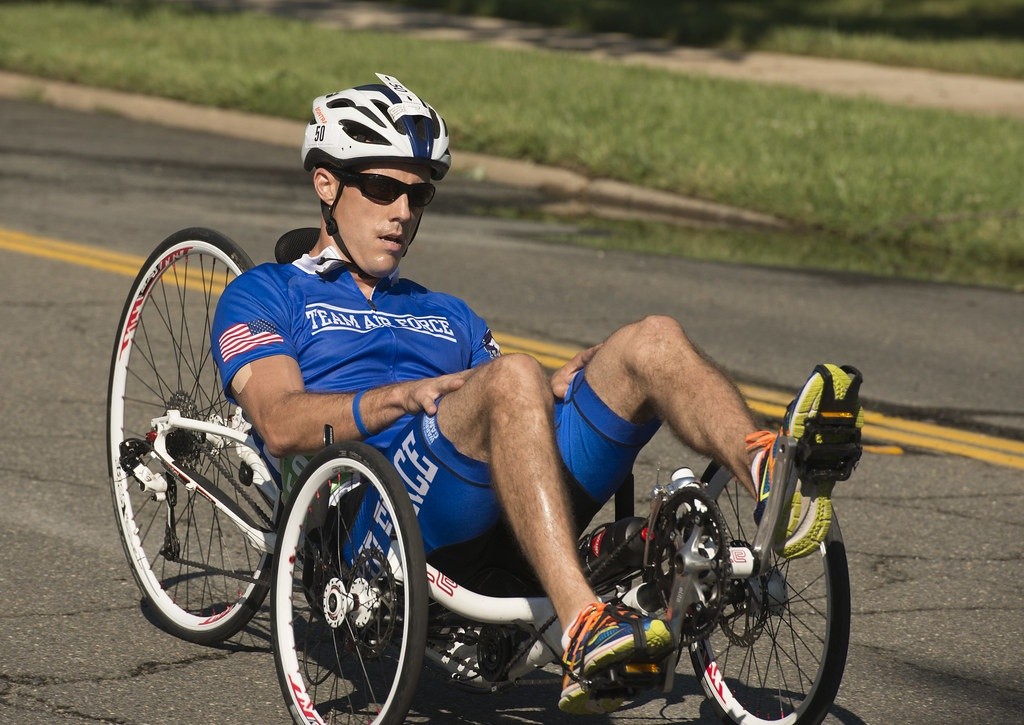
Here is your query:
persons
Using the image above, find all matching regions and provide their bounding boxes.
[211,70,865,716]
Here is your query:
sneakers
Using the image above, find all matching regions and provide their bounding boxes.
[557,603,671,715]
[741,363,865,562]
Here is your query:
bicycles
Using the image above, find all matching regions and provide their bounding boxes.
[101,222,853,725]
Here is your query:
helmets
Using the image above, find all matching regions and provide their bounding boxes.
[300,72,452,180]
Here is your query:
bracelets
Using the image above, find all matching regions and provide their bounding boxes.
[352,388,380,437]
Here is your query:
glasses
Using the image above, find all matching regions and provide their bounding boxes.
[316,164,437,210]
